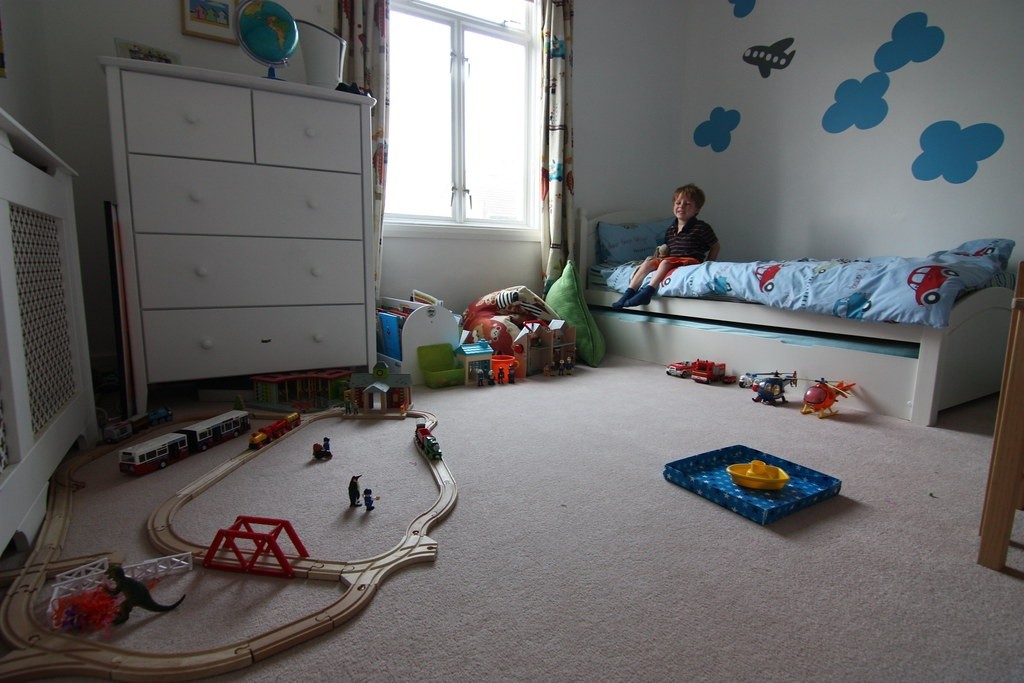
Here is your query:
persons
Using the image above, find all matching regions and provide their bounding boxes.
[612,183,720,309]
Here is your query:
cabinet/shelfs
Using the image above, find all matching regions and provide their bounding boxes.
[100,57,377,414]
[0,105,99,558]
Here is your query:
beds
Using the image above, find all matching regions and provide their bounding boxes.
[577,207,1015,427]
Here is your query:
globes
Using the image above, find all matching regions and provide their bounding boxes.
[231,0,299,81]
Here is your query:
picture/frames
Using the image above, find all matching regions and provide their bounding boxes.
[181,0,240,45]
[114,37,182,66]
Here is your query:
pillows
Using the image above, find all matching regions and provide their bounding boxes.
[546,260,605,367]
[597,217,676,264]
